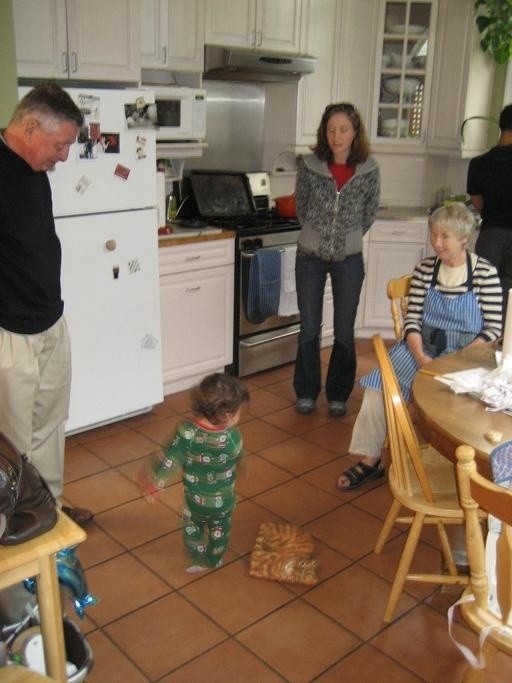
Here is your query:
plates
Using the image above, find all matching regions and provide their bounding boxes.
[20,631,82,680]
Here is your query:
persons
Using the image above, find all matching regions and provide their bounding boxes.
[295,101,383,418]
[333,202,503,491]
[140,371,249,572]
[465,100,512,329]
[2,82,95,527]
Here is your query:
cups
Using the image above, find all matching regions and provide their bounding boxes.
[383,52,427,68]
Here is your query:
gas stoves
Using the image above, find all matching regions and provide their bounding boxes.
[200,207,302,235]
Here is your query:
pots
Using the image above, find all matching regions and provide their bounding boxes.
[273,195,300,218]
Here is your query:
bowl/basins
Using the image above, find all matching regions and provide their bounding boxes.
[381,117,411,137]
[384,77,423,97]
[387,23,425,34]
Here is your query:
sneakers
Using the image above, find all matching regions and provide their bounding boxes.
[62,506,93,524]
[329,401,347,417]
[296,398,315,414]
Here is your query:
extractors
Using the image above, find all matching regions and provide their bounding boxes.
[202,42,317,85]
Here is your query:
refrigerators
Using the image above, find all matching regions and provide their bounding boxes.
[14,83,167,439]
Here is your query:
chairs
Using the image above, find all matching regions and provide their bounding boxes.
[364,332,489,624]
[448,444,511,682]
[389,270,416,343]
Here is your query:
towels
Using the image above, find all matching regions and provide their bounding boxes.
[246,248,281,323]
[279,245,305,317]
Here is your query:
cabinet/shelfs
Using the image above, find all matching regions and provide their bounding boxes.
[370,1,439,157]
[131,0,205,88]
[427,1,499,160]
[10,1,139,90]
[263,0,387,150]
[199,0,301,54]
[155,235,235,398]
[350,222,478,344]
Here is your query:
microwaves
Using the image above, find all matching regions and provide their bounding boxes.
[143,84,208,144]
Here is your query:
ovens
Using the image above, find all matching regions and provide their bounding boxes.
[227,230,325,380]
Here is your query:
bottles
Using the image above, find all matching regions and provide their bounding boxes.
[167,190,178,221]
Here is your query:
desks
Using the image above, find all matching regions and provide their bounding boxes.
[0,502,87,683]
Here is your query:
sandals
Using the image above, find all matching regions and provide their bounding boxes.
[336,456,385,490]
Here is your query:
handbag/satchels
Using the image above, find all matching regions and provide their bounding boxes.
[1,431,57,545]
